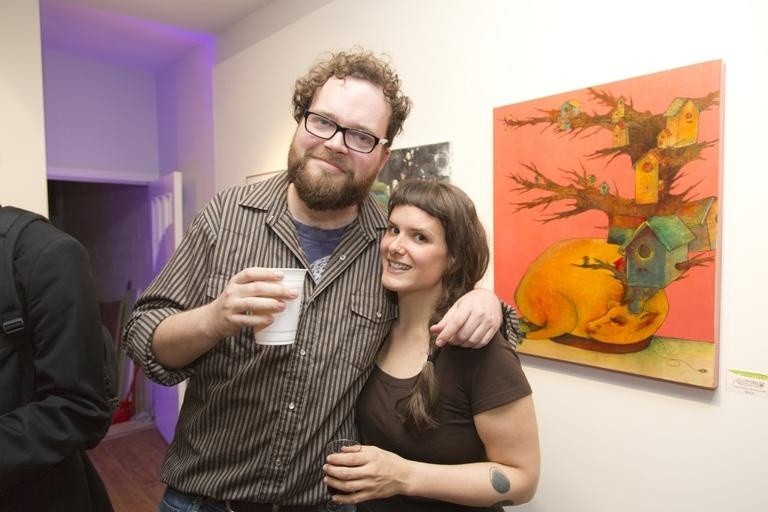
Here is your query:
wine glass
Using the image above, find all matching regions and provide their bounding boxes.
[325,438,360,512]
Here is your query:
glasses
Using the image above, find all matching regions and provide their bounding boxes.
[300,111,392,156]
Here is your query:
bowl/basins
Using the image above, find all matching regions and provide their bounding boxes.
[110,400,134,423]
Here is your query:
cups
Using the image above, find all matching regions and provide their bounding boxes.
[249,267,307,345]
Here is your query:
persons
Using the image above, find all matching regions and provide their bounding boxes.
[0,204,117,511]
[124,45,529,512]
[321,177,542,512]
